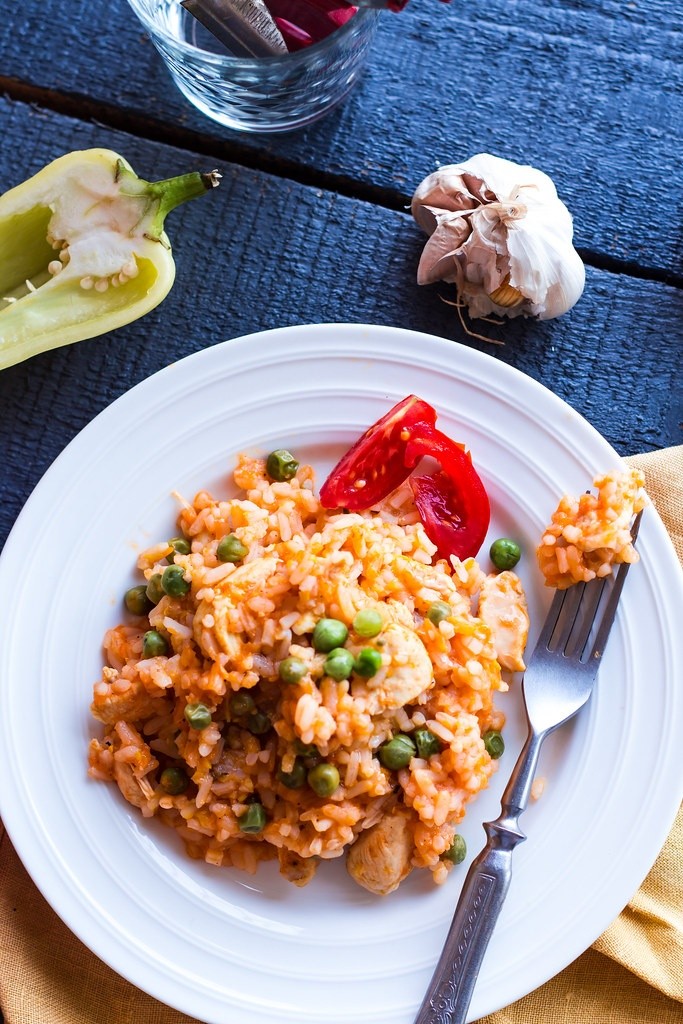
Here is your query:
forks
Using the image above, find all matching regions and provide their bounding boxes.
[414,492,642,1024]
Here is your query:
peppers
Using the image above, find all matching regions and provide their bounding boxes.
[0,146,223,380]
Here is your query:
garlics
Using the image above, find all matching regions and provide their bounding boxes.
[411,151,586,323]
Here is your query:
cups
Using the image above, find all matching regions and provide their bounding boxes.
[130,0,379,135]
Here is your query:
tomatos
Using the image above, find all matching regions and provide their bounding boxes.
[315,394,491,568]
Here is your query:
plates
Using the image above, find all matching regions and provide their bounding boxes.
[0,323,683,1024]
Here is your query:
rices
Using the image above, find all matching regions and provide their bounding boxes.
[86,448,647,900]
